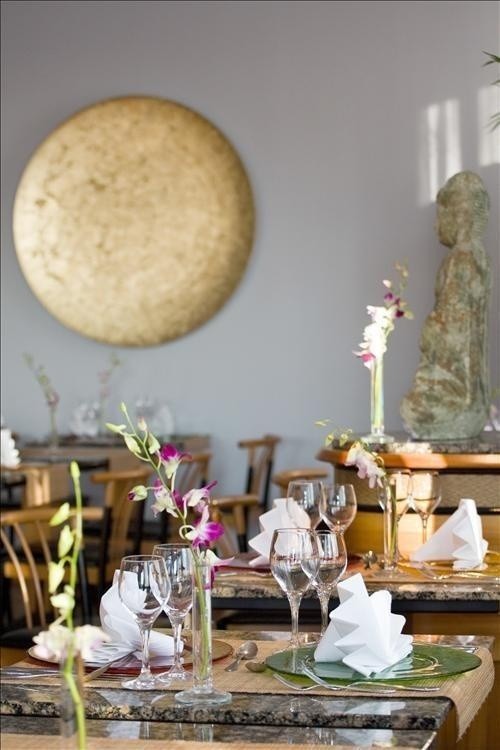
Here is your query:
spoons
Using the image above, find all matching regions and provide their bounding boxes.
[223,641,259,675]
[362,550,378,571]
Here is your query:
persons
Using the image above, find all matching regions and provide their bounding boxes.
[400,172,491,451]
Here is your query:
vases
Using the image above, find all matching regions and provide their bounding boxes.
[363,349,390,440]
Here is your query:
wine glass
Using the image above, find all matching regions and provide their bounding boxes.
[149,542,198,685]
[377,467,412,575]
[313,529,348,645]
[407,471,442,544]
[118,554,172,694]
[318,483,360,533]
[269,527,325,670]
[285,479,326,529]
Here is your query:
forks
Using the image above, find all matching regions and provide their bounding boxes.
[273,673,396,695]
[297,659,442,692]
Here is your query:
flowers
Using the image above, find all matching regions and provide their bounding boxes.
[21,347,62,448]
[92,350,122,432]
[350,252,417,442]
[111,394,230,683]
